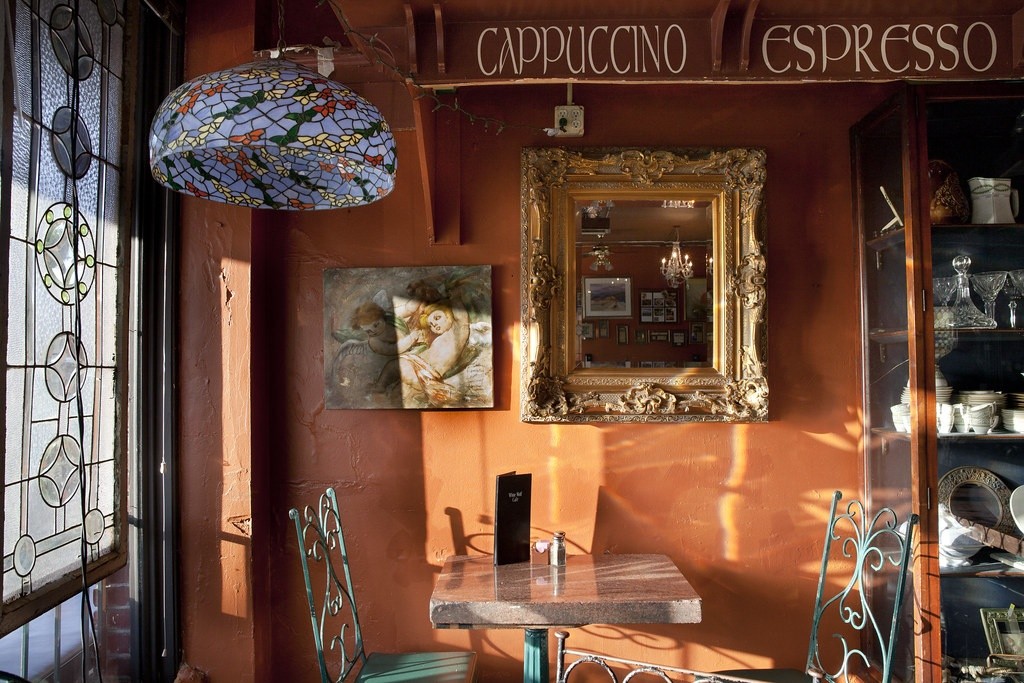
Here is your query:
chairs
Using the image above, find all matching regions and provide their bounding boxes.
[289,488,480,683]
[696,490,919,683]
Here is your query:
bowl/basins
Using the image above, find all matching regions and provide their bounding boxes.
[1010,486,1023,535]
[889,402,954,433]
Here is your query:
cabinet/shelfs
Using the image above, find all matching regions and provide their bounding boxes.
[849,78,1024,683]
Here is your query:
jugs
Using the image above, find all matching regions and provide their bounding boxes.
[968,176,1020,224]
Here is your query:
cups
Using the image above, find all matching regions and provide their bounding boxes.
[934,307,955,330]
[953,400,1000,434]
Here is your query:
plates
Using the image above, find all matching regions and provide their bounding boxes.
[900,387,953,403]
[954,390,1024,434]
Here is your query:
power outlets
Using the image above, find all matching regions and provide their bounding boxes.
[554,106,584,137]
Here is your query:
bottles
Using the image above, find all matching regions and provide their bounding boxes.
[952,255,998,329]
[549,531,567,567]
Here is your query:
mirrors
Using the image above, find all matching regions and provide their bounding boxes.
[519,144,770,423]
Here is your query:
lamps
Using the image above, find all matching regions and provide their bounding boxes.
[149,1,397,210]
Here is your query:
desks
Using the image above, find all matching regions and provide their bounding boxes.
[429,553,702,683]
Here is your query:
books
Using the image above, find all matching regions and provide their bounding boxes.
[494,560,532,602]
[493,470,532,566]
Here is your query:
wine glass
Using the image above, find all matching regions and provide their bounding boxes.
[933,270,1024,330]
[906,331,959,386]
